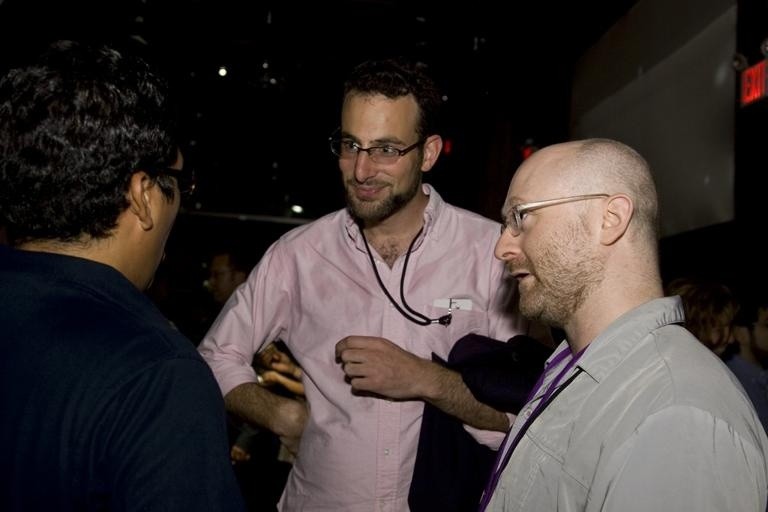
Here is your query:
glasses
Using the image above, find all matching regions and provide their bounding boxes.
[501,194,609,237]
[328,126,421,164]
[154,165,197,194]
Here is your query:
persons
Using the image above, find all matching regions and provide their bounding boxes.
[194,51,559,511]
[199,227,767,511]
[1,16,253,510]
[468,137,768,510]
